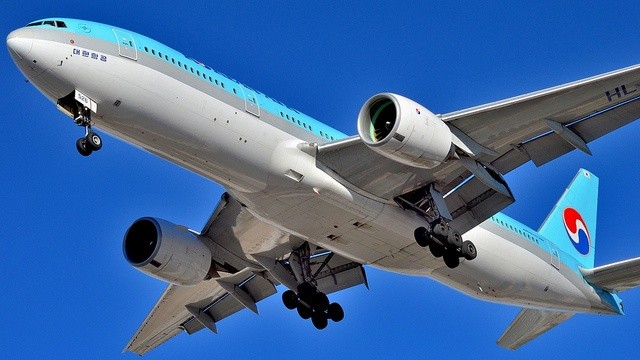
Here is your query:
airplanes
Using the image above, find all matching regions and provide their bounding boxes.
[6,14,640,357]
